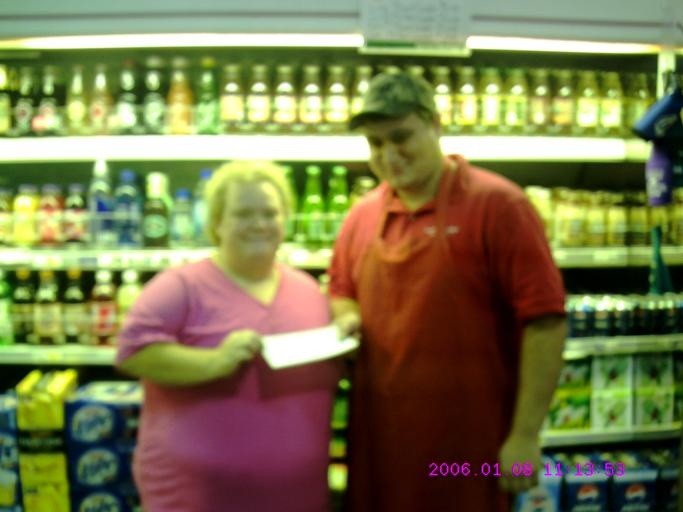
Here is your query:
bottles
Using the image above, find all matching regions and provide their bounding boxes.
[0,160,210,347]
[524,186,682,249]
[283,164,374,252]
[0,54,653,139]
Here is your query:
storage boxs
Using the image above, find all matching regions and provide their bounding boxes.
[521,352,680,512]
[0,367,144,512]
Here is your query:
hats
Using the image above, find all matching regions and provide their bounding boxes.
[345,68,437,132]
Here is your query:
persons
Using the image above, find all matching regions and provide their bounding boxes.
[327,73,567,510]
[116,159,333,512]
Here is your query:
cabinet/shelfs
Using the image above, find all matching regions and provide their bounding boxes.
[0,33,683,512]
